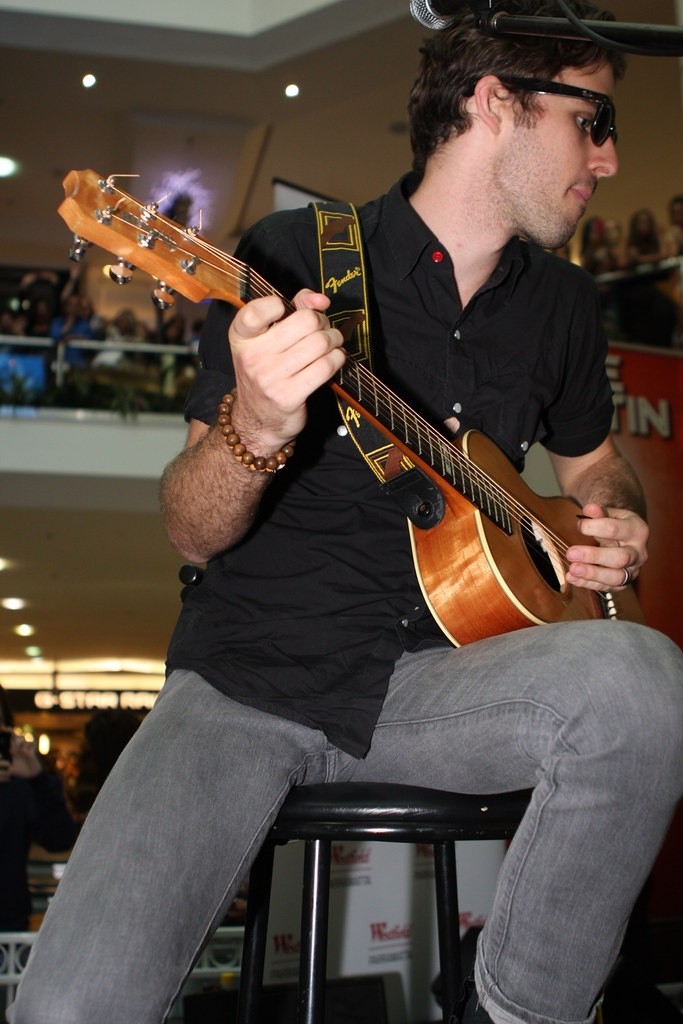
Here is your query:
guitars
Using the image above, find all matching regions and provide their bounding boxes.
[58,163,649,648]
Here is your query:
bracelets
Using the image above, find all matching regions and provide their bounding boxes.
[216,387,296,479]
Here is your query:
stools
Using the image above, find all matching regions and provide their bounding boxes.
[237,782,536,1024]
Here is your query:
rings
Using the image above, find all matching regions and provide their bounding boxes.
[622,568,629,585]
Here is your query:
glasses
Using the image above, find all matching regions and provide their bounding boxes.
[463,75,618,147]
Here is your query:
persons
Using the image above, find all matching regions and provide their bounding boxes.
[557,197,683,345]
[0,269,203,410]
[0,686,80,1024]
[10,1,683,1024]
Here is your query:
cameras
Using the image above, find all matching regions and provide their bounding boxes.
[0,731,13,766]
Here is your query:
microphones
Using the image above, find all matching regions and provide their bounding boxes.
[409,0,511,31]
